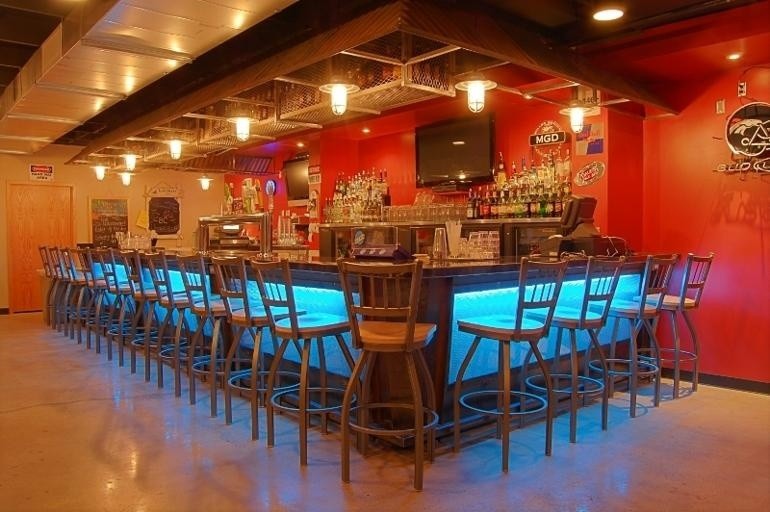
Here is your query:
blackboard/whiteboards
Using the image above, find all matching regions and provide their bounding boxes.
[90,194,130,247]
[146,182,182,240]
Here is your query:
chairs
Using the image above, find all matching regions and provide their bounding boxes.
[48,246,81,340]
[75,245,118,355]
[336,257,439,493]
[38,244,64,327]
[632,250,716,398]
[209,251,307,442]
[144,250,192,399]
[519,255,626,445]
[249,254,363,466]
[119,248,187,381]
[174,249,243,418]
[583,254,681,430]
[60,247,101,347]
[95,247,155,368]
[451,258,570,475]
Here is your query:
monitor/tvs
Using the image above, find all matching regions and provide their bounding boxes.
[414,112,496,188]
[282,158,309,200]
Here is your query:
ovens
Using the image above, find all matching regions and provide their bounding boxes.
[349,227,411,261]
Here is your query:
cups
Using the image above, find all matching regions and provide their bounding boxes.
[433,228,447,260]
[297,245,309,261]
[383,191,465,221]
[456,231,499,258]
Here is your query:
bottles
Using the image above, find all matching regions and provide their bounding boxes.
[325,165,390,223]
[468,192,474,219]
[483,192,490,218]
[506,190,517,217]
[476,194,482,217]
[554,148,564,177]
[522,188,533,218]
[491,151,545,188]
[490,191,500,219]
[563,148,571,176]
[515,189,524,217]
[562,185,571,211]
[546,154,556,180]
[536,188,546,220]
[498,193,510,218]
[555,188,563,219]
[546,192,554,217]
[530,190,537,218]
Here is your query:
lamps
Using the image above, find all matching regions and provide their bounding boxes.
[88,159,112,181]
[318,74,361,117]
[558,87,599,134]
[115,170,137,187]
[161,132,190,160]
[226,108,260,142]
[454,70,497,115]
[117,147,143,171]
[195,174,216,191]
[590,0,630,23]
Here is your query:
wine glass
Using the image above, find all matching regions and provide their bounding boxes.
[151,233,158,254]
